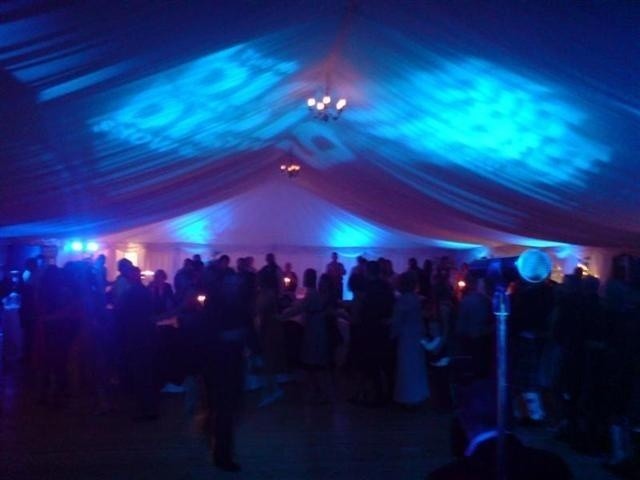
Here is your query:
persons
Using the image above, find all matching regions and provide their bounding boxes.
[2,249,639,479]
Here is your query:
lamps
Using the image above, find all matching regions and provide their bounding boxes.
[280,146,300,179]
[307,65,347,124]
